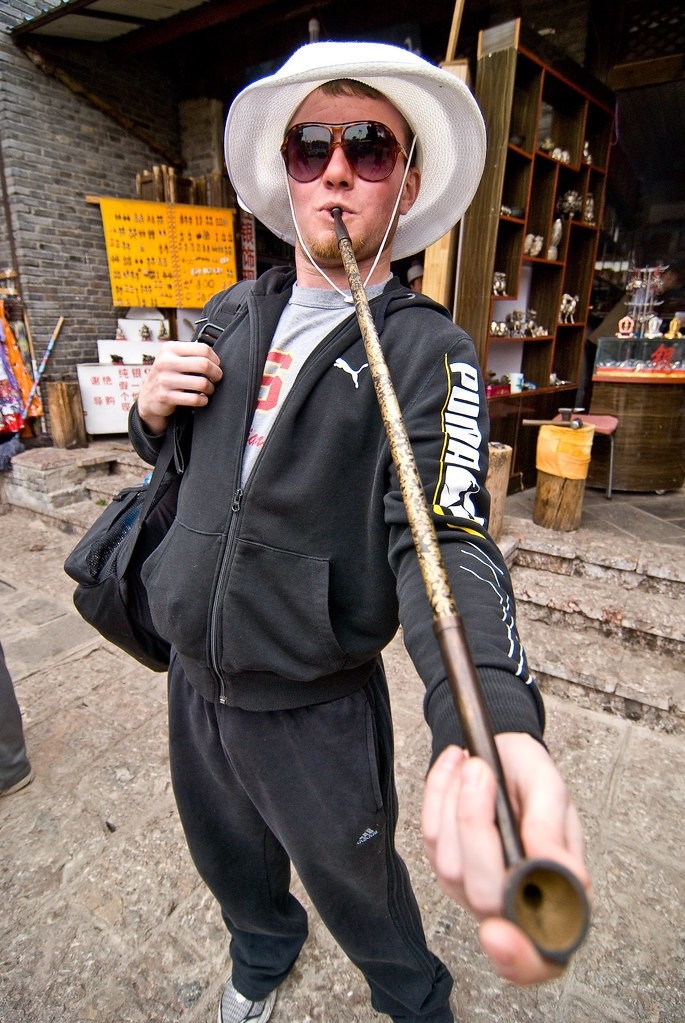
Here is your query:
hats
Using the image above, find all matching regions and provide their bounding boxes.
[222,43,487,262]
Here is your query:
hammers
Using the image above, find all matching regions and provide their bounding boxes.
[523,418,584,429]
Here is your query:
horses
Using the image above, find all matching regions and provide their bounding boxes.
[558,293,580,324]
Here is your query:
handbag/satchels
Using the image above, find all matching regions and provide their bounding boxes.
[64,280,251,670]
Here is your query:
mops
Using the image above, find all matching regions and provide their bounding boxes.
[0,315,64,474]
[22,307,54,448]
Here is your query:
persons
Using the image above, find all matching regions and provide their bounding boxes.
[348,139,383,169]
[581,262,685,414]
[299,145,314,174]
[0,642,35,796]
[127,43,590,1023]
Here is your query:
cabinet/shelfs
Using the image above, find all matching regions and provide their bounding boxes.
[454,51,612,500]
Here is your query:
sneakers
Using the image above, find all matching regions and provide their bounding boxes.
[217,972,277,1023]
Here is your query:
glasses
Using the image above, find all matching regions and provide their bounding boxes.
[280,120,409,183]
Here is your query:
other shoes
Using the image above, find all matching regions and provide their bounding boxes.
[0,767,32,797]
